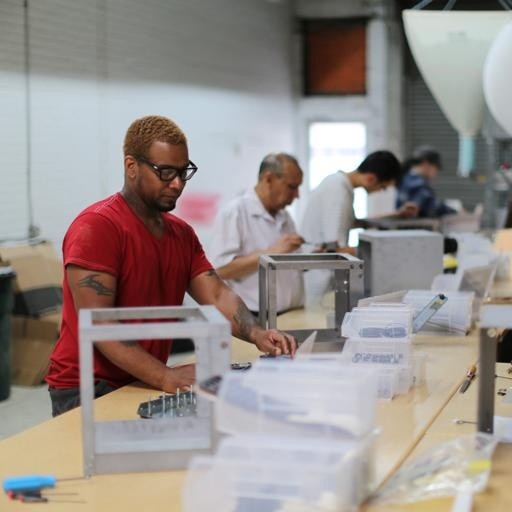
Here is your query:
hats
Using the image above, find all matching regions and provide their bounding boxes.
[414,150,443,169]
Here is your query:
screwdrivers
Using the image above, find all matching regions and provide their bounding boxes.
[7,490,80,497]
[3,474,95,491]
[18,497,81,505]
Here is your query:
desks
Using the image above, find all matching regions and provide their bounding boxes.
[0,229,512,512]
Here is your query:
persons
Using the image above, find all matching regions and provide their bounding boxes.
[293,150,403,298]
[203,152,326,322]
[44,113,300,414]
[395,146,459,219]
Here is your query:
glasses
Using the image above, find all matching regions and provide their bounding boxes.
[136,154,197,182]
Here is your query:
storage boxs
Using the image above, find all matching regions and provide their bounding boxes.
[0,240,65,386]
